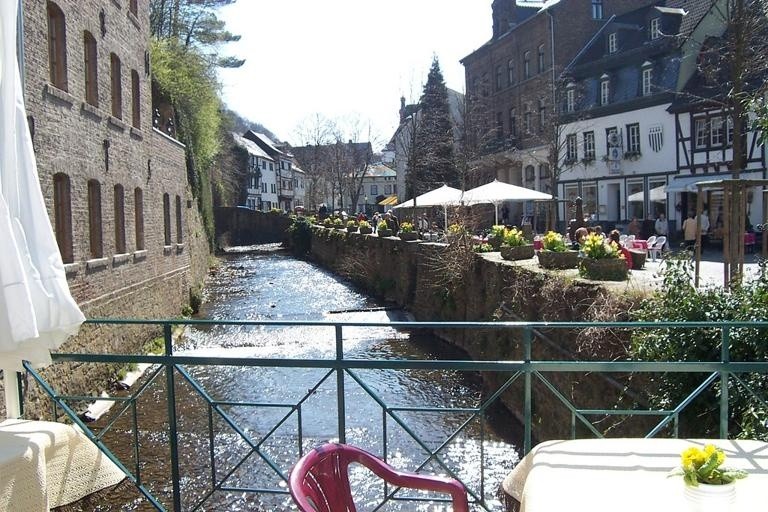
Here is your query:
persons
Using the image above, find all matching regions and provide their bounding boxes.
[293,207,712,255]
[500,203,511,223]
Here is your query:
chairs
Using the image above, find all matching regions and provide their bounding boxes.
[619,234,667,263]
[288,441,470,512]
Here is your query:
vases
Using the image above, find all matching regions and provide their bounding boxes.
[675,475,737,510]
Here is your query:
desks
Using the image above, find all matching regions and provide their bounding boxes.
[0,417,80,512]
[521,437,767,510]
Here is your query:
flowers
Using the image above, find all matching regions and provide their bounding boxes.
[492,223,623,260]
[307,215,413,233]
[666,444,748,484]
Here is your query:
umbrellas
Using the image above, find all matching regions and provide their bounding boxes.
[0,0,88,422]
[455,177,555,223]
[390,184,466,235]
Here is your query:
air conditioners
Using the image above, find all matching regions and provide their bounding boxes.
[608,147,623,160]
